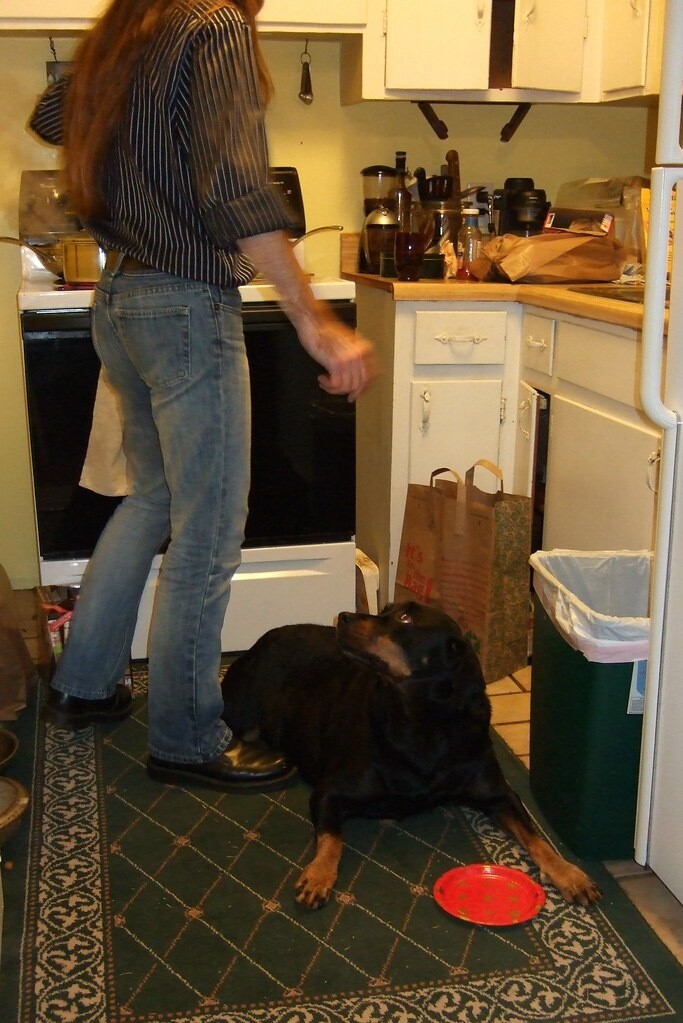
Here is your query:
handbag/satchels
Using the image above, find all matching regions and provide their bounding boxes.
[474,230,622,284]
[395,460,532,685]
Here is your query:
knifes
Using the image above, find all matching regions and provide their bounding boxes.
[413,150,462,200]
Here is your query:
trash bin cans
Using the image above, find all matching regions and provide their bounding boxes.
[528,549,654,860]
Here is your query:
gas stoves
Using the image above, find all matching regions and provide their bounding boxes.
[16,170,358,317]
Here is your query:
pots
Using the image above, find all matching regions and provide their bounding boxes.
[254,225,343,280]
[0,222,107,285]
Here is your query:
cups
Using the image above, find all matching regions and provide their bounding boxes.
[394,221,425,281]
[409,197,462,258]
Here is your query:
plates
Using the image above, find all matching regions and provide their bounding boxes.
[433,864,546,925]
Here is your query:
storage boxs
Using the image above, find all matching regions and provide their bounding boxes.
[638,187,676,286]
[543,207,615,238]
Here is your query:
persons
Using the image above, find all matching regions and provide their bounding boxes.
[29,0,377,788]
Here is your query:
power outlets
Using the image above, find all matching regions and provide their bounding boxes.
[467,182,494,228]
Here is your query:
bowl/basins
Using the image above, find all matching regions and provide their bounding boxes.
[0,775,30,850]
[0,727,19,767]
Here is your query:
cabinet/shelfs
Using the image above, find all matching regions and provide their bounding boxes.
[339,0,593,107]
[512,302,669,550]
[593,0,683,108]
[355,283,530,603]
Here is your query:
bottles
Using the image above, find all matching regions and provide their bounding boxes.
[492,178,551,238]
[457,208,482,280]
[388,150,411,228]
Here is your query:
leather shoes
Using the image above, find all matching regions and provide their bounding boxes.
[147,742,297,794]
[46,683,131,728]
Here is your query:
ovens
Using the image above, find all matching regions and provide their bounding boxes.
[20,312,358,663]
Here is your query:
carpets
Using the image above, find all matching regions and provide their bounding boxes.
[0,651,683,1023]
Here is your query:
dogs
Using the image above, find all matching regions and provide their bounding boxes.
[221,601,602,910]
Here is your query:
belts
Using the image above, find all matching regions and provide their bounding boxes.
[104,249,151,272]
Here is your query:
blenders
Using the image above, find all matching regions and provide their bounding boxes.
[358,164,409,277]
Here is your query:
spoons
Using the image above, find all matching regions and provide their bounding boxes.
[456,186,486,202]
